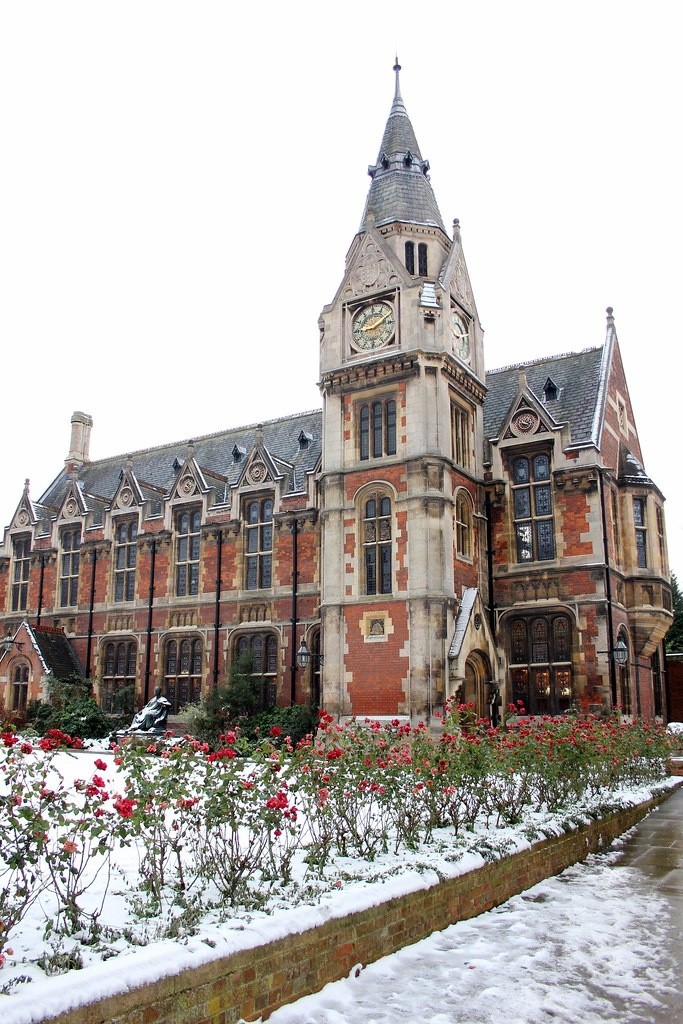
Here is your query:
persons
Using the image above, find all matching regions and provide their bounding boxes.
[126,686,171,732]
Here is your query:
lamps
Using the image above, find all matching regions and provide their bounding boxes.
[4,628,13,654]
[295,635,324,669]
[612,630,628,666]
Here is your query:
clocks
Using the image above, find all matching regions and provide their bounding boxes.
[449,310,473,365]
[347,298,397,353]
